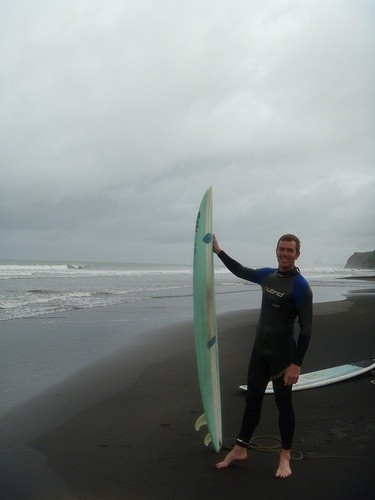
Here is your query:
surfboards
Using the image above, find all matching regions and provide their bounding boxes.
[191,186,225,452]
[239,358,375,394]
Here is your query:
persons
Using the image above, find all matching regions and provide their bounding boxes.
[213,233,313,477]
